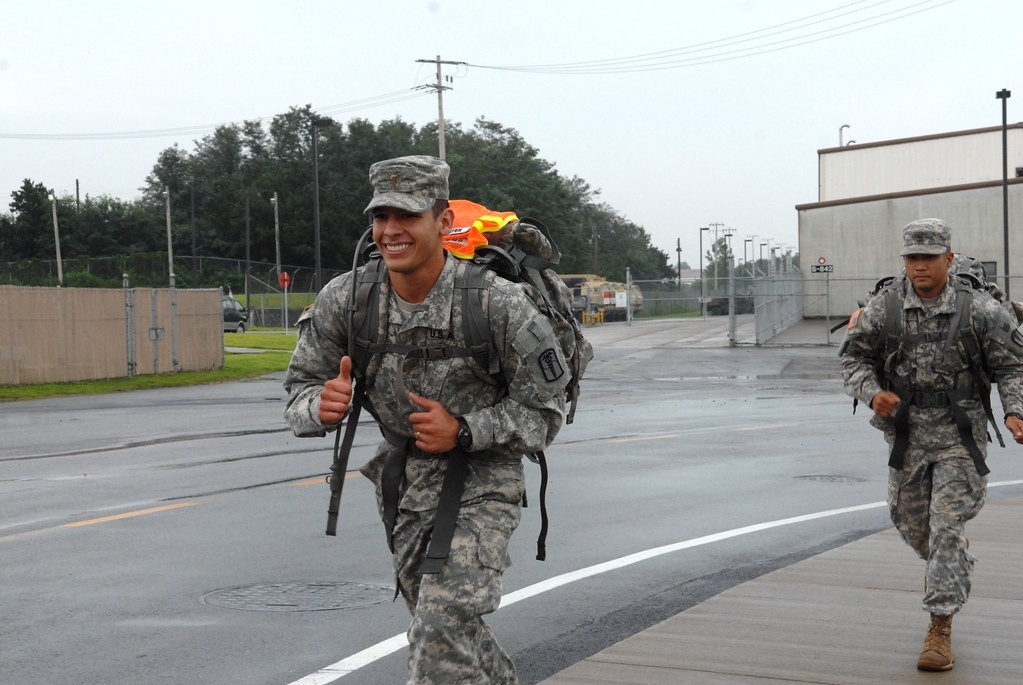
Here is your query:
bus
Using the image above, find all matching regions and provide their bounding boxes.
[555,273,643,320]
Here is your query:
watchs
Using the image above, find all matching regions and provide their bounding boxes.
[455,415,472,452]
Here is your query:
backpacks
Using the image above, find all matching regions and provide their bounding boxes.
[361,201,594,403]
[859,254,987,390]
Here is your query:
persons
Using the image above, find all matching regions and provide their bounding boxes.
[837,218,1023,672]
[281,156,573,685]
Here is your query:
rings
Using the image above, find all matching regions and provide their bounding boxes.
[415,432,421,440]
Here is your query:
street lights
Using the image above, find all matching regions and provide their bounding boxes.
[846,140,857,148]
[47,190,64,285]
[744,239,752,295]
[699,227,710,314]
[839,125,850,148]
[723,234,734,295]
[995,88,1013,300]
[162,184,176,288]
[271,190,283,283]
[759,243,767,277]
[771,246,781,275]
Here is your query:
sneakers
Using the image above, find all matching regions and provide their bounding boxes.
[917,613,954,670]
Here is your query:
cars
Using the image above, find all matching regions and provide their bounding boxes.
[222,297,250,335]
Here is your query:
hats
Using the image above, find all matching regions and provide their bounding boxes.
[898,217,951,256]
[362,154,450,215]
